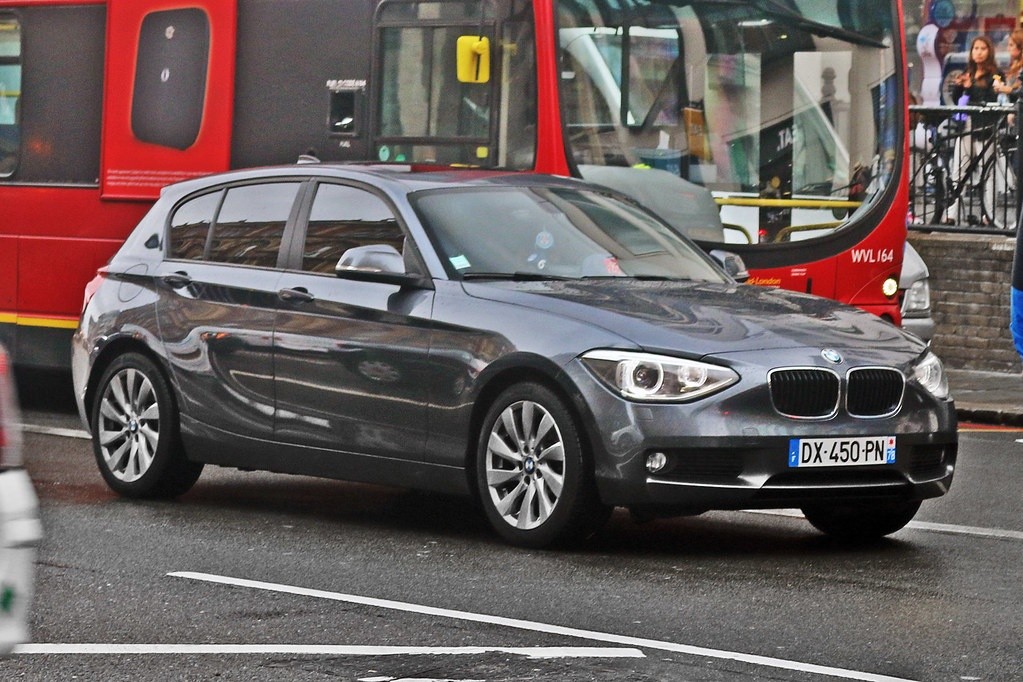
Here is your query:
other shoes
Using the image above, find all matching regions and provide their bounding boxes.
[1015,438,1023,443]
[943,218,956,226]
[908,213,923,225]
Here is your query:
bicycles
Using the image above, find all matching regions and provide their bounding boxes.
[909,100,1019,242]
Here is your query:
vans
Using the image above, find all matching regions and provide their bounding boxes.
[546,18,938,343]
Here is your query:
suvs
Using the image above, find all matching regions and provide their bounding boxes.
[69,154,962,554]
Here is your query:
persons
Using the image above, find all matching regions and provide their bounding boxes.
[992,29,1023,230]
[908,35,1006,227]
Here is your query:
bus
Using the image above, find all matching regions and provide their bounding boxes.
[0,0,918,373]
